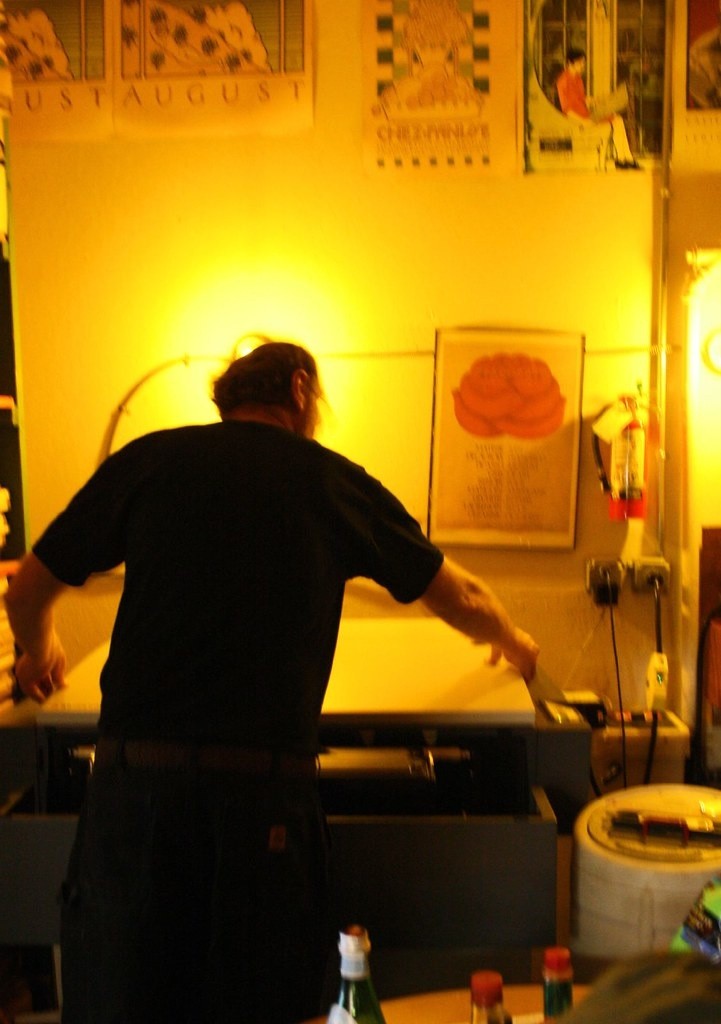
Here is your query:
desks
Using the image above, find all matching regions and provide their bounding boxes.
[0,662,595,1009]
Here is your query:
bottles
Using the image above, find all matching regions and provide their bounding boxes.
[668,873,721,962]
[469,970,513,1024]
[542,946,575,1024]
[326,923,389,1024]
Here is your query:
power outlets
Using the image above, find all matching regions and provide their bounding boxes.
[633,557,671,595]
[584,557,626,592]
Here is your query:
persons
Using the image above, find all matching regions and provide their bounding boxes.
[5,340,538,1024]
